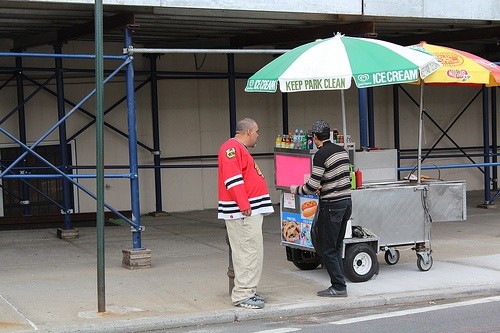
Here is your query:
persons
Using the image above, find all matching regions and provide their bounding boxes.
[290,120,352,297]
[217,118,275,309]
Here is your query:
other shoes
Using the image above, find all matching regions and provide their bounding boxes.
[236,297,265,309]
[250,293,266,302]
[317,286,347,296]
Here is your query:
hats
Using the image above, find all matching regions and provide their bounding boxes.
[308,119,330,133]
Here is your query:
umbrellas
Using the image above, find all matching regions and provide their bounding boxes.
[405,41,500,184]
[244,32,442,151]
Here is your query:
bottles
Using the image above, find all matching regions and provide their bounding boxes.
[347,135,352,148]
[332,128,339,139]
[356,169,362,187]
[350,172,356,189]
[276,128,313,153]
